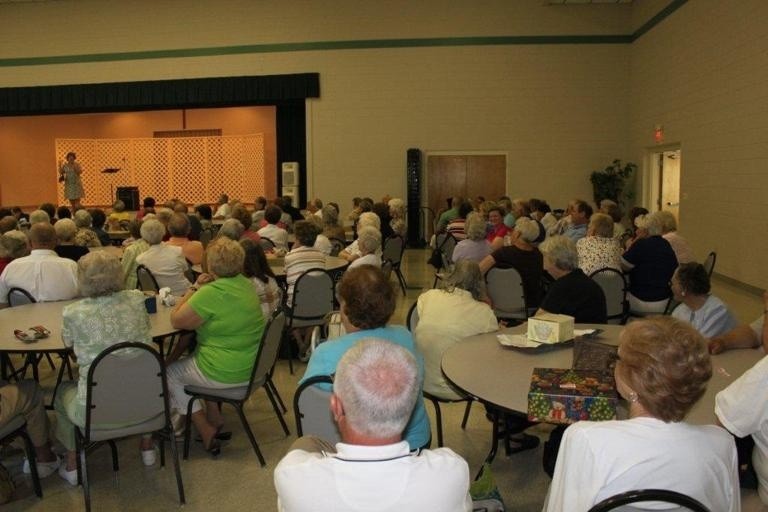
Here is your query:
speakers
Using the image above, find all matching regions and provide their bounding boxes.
[116,187,140,211]
[282,162,300,187]
[282,187,300,209]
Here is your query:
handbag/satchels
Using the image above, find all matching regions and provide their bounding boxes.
[58,175,65,182]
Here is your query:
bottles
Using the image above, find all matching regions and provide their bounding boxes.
[144,291,157,315]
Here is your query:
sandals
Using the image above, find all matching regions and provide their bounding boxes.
[508,432,541,453]
[194,425,232,456]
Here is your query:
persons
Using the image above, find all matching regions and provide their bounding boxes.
[669,263,738,340]
[0,193,696,487]
[708,289,768,356]
[58,152,85,208]
[273,338,474,512]
[539,314,741,512]
[715,354,768,512]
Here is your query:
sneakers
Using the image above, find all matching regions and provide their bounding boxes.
[23,455,78,487]
[141,448,158,466]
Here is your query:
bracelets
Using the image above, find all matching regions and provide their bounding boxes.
[763,310,768,313]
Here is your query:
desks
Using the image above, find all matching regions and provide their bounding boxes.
[440,324,767,482]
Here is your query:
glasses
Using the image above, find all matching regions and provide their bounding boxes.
[608,349,623,364]
